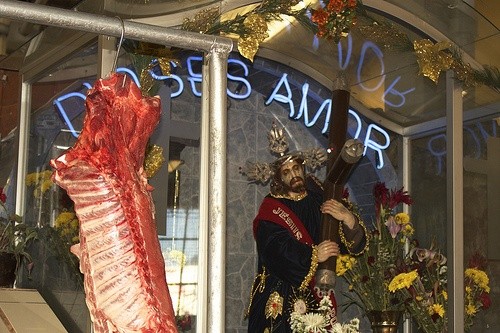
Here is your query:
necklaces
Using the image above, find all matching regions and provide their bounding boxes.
[267,185,309,202]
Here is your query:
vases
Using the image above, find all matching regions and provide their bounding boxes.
[0,251,17,287]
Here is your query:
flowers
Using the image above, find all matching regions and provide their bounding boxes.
[0,187,39,280]
[25,170,85,292]
[334,180,491,333]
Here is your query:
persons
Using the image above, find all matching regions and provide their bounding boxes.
[245,150,371,333]
